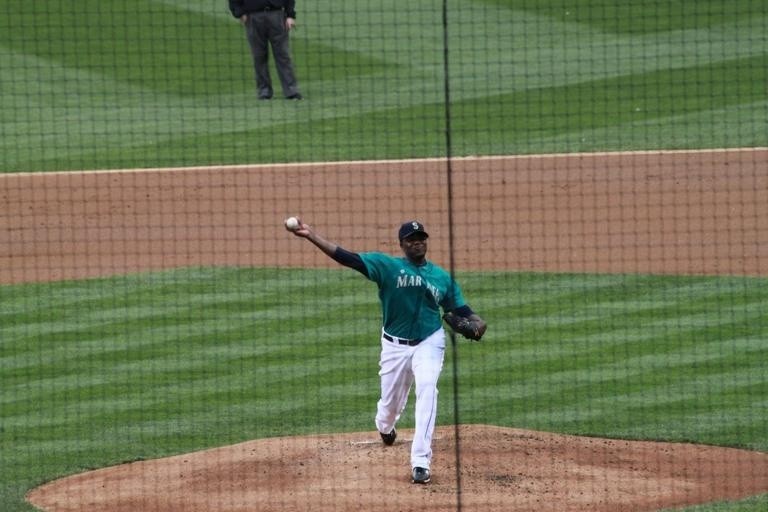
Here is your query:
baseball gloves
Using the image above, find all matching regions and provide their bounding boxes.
[443,312,484,342]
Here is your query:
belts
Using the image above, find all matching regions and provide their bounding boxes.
[383,334,420,346]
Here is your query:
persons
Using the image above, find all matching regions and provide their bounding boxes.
[228,1,303,99]
[282,211,488,483]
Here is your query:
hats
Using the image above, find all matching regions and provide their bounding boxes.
[398,222,427,240]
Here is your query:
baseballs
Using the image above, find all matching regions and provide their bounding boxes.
[286,217,298,230]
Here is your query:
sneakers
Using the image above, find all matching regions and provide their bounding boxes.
[412,467,430,484]
[380,427,397,446]
